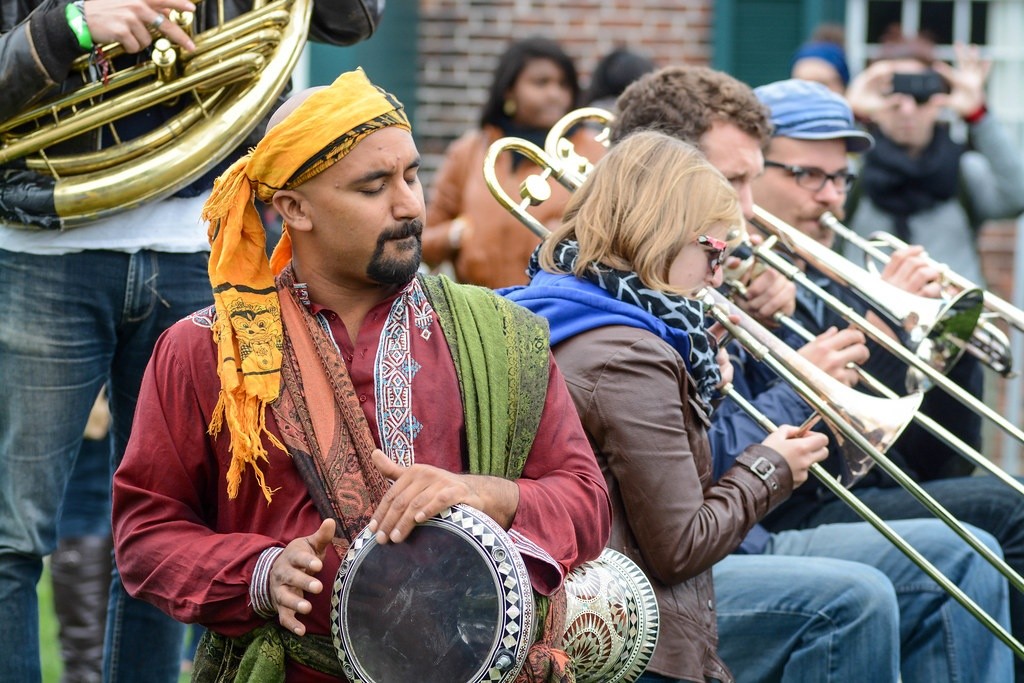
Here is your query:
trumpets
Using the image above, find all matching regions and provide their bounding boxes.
[816,209,1023,377]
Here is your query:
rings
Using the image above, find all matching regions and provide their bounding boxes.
[153,14,165,28]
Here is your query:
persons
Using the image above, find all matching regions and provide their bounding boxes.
[741,73,1024,682]
[491,130,902,683]
[605,63,1016,683]
[110,66,614,683]
[420,25,1023,491]
[2,0,384,683]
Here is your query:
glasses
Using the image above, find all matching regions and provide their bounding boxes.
[765,161,857,193]
[691,232,727,275]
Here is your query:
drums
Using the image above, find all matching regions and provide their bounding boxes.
[327,500,661,683]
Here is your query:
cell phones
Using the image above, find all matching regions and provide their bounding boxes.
[891,71,944,102]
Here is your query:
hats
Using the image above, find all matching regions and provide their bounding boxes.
[754,78,876,154]
[789,41,849,87]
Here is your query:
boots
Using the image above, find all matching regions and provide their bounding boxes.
[51,536,110,683]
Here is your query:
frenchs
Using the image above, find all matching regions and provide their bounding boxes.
[0,0,318,238]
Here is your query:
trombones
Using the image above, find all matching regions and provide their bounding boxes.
[540,105,1024,497]
[480,135,1024,663]
